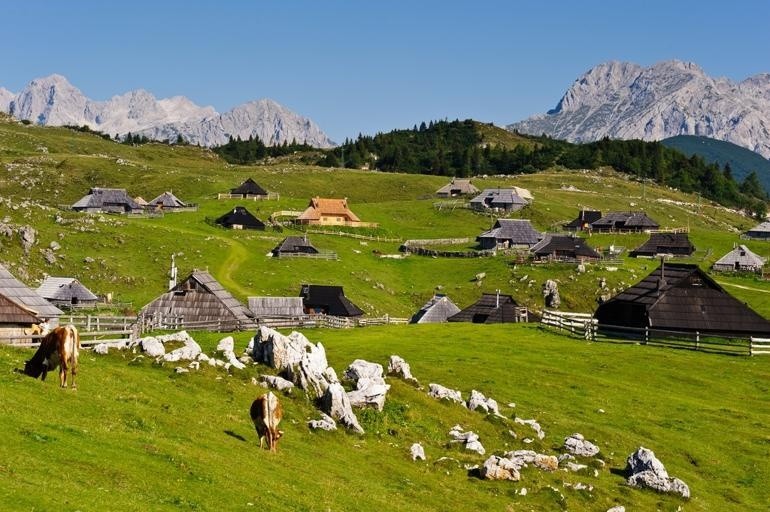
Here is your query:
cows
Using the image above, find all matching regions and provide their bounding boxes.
[250,391,283,452]
[24,324,79,393]
